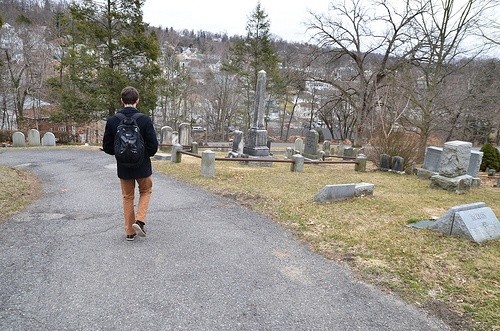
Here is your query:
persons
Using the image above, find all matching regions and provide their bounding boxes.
[104,85,158,241]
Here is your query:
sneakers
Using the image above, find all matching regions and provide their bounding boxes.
[126,234,137,240]
[131,220,147,238]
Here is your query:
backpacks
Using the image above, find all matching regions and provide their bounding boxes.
[114,112,148,164]
